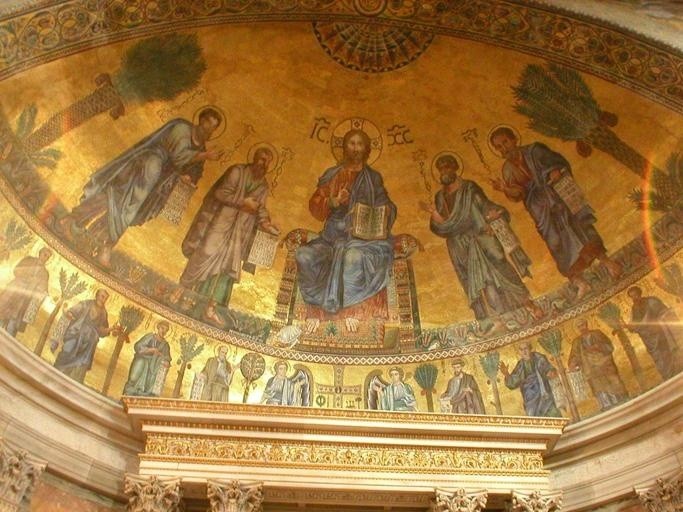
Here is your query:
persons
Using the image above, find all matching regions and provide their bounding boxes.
[66,108,226,257]
[485,127,607,284]
[415,154,536,321]
[295,129,399,266]
[0,248,682,421]
[177,148,282,306]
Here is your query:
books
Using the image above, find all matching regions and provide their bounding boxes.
[350,201,389,242]
